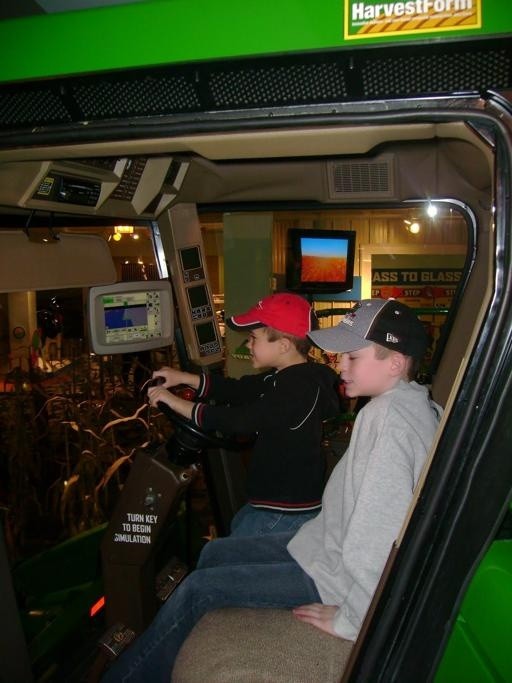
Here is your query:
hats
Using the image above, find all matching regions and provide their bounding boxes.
[232,292,318,340]
[306,297,428,364]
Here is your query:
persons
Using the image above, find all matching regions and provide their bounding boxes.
[147,293,341,539]
[100,297,446,683]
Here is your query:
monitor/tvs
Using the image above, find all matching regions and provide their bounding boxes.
[286,228,356,294]
[89,280,173,356]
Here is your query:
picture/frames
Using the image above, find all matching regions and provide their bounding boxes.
[360,243,468,299]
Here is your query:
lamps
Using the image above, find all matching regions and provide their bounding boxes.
[402,217,422,235]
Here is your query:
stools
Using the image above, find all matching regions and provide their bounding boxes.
[172,607,351,683]
